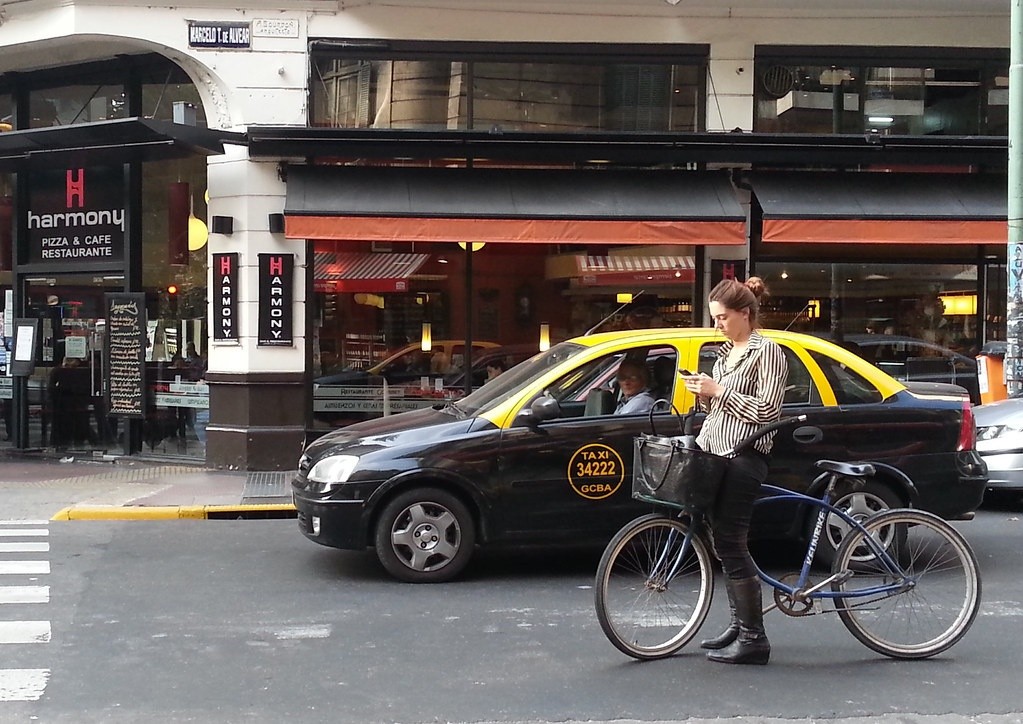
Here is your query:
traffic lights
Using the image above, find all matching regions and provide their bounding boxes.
[168,285,178,311]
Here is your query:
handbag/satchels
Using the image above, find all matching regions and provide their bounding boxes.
[638,398,695,505]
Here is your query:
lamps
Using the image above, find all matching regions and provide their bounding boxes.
[864,132,881,144]
[269,213,286,233]
[808,297,820,318]
[421,320,433,353]
[539,320,551,352]
[936,291,977,315]
[188,193,209,251]
[211,215,233,234]
[616,293,633,304]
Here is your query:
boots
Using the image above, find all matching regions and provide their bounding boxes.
[700,567,740,650]
[706,574,771,665]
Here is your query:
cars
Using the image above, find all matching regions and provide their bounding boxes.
[62,324,90,344]
[313,338,513,424]
[369,340,566,418]
[291,329,989,585]
[969,389,1022,488]
[559,348,973,408]
[785,335,982,406]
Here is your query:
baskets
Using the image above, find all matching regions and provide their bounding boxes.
[631,435,729,513]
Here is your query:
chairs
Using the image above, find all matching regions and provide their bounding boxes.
[654,355,675,410]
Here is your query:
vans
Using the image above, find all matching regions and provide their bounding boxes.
[96,322,106,335]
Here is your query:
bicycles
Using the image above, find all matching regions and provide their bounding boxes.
[592,407,982,661]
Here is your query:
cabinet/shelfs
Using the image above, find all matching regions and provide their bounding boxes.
[340,338,386,371]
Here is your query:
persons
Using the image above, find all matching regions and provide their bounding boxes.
[144,340,203,447]
[485,360,506,383]
[431,353,461,375]
[615,359,658,417]
[44,356,99,449]
[679,276,791,666]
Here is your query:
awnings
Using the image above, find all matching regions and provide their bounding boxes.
[753,170,1009,244]
[282,164,747,248]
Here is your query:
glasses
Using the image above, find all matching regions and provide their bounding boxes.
[617,374,643,383]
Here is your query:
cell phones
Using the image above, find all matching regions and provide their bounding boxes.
[679,369,692,376]
